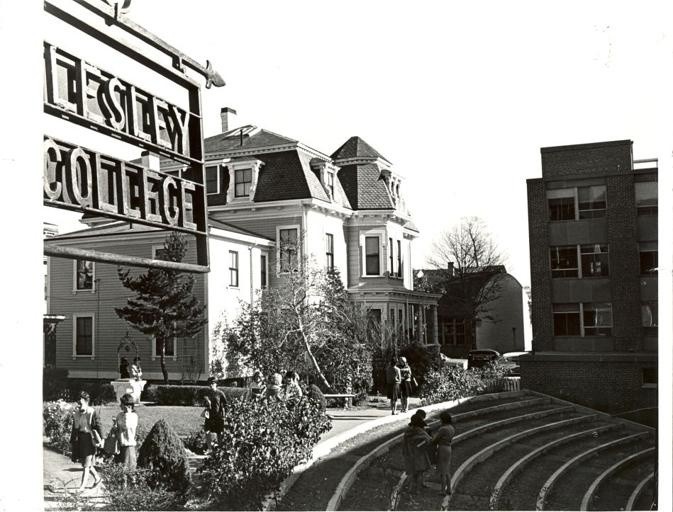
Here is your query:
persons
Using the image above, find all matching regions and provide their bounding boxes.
[129,357,143,381]
[401,409,456,496]
[397,356,413,412]
[202,377,229,455]
[120,357,130,378]
[112,394,139,488]
[246,371,303,402]
[386,357,401,415]
[70,390,108,492]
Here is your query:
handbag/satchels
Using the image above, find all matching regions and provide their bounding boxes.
[103,427,120,455]
[199,408,210,420]
[88,409,101,448]
